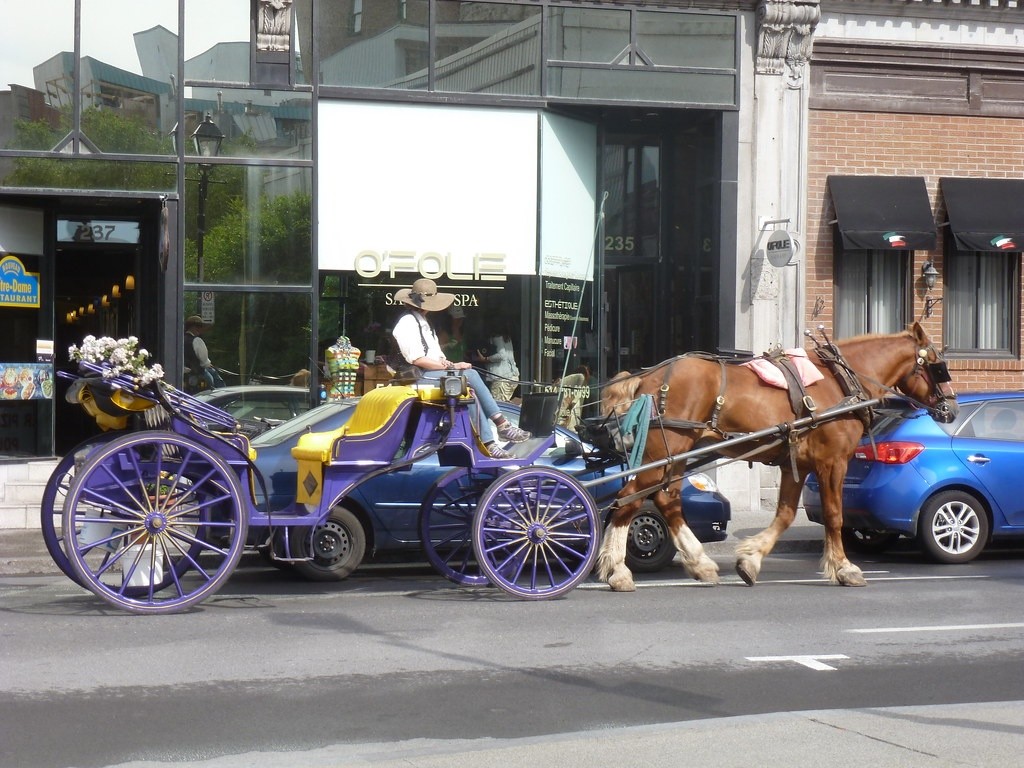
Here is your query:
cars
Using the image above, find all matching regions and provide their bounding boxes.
[796,385,1024,564]
[176,375,322,429]
[239,393,734,579]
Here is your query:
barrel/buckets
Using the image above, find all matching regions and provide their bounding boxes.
[118,544,166,588]
[79,508,130,552]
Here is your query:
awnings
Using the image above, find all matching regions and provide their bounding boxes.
[823,175,935,251]
[936,178,1024,254]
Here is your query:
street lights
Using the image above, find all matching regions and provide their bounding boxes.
[190,108,225,319]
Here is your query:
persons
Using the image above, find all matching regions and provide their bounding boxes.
[184,314,225,390]
[389,277,529,461]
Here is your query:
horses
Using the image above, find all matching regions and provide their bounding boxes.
[595,321,962,592]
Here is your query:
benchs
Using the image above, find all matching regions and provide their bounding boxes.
[83,369,492,464]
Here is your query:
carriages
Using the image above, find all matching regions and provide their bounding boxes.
[41,314,959,614]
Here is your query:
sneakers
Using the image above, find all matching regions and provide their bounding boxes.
[497,423,531,443]
[487,445,517,461]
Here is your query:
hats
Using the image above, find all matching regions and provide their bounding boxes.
[185,315,213,329]
[393,278,455,311]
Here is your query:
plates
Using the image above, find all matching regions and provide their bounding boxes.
[0,368,53,400]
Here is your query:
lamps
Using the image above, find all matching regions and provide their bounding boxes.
[921,257,941,294]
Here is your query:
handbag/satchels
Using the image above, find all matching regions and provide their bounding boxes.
[474,341,497,357]
